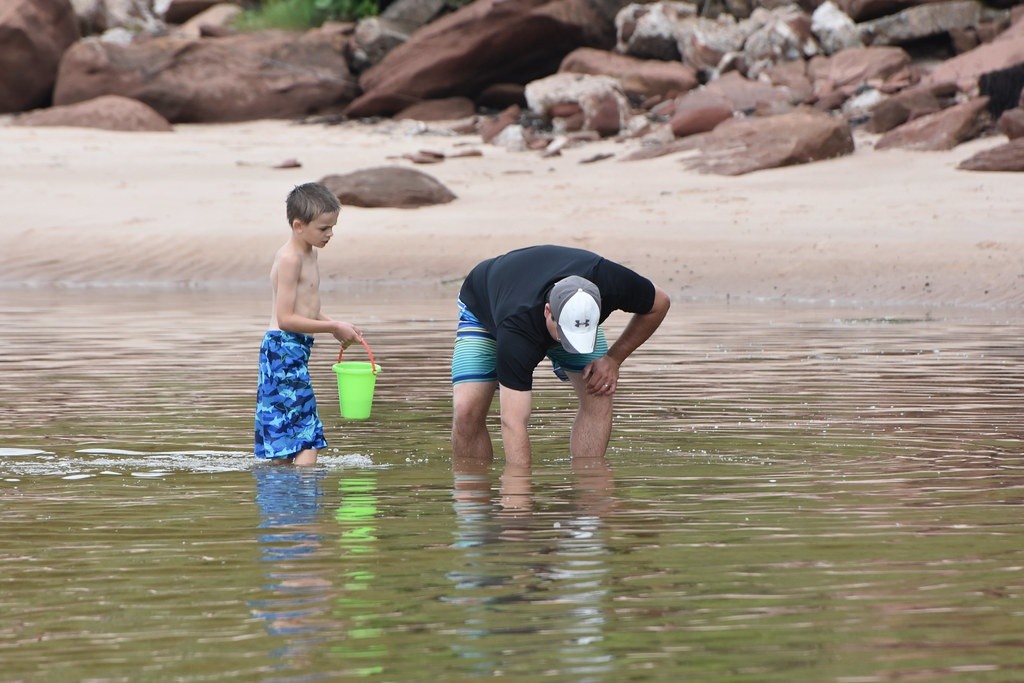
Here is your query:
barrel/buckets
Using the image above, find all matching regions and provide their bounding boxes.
[332,336,383,419]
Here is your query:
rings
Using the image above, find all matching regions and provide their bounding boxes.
[604,384,611,391]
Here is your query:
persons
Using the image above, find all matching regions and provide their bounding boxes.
[453,244,670,481]
[255,184,365,467]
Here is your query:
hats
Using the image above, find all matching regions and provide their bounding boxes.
[550,276,601,354]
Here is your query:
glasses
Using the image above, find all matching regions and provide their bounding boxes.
[555,327,561,342]
